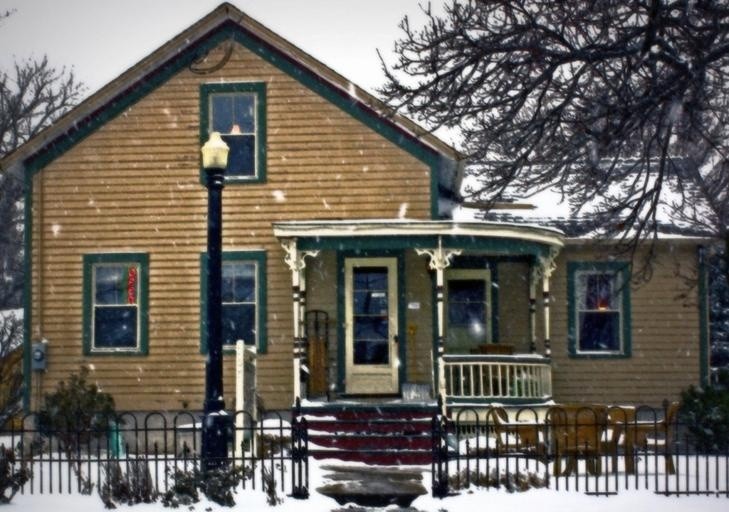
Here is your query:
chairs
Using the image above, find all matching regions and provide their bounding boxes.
[470,343,522,396]
[489,400,679,476]
